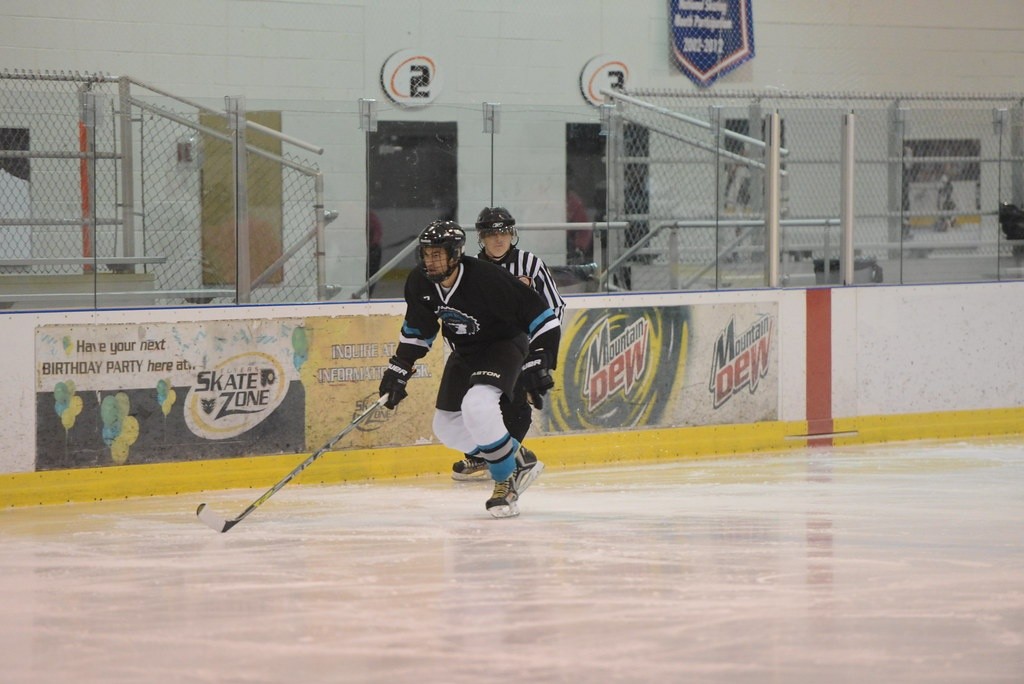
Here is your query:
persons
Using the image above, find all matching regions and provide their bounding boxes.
[379,220,562,518]
[451,207,565,482]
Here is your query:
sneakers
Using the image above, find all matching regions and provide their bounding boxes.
[452,457,492,480]
[485,474,520,516]
[514,443,545,495]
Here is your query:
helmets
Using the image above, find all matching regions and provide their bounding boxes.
[475,206,515,227]
[418,220,466,284]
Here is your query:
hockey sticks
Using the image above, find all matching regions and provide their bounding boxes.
[196,368,418,533]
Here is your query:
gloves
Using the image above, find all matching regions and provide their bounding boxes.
[521,351,554,410]
[379,355,412,409]
